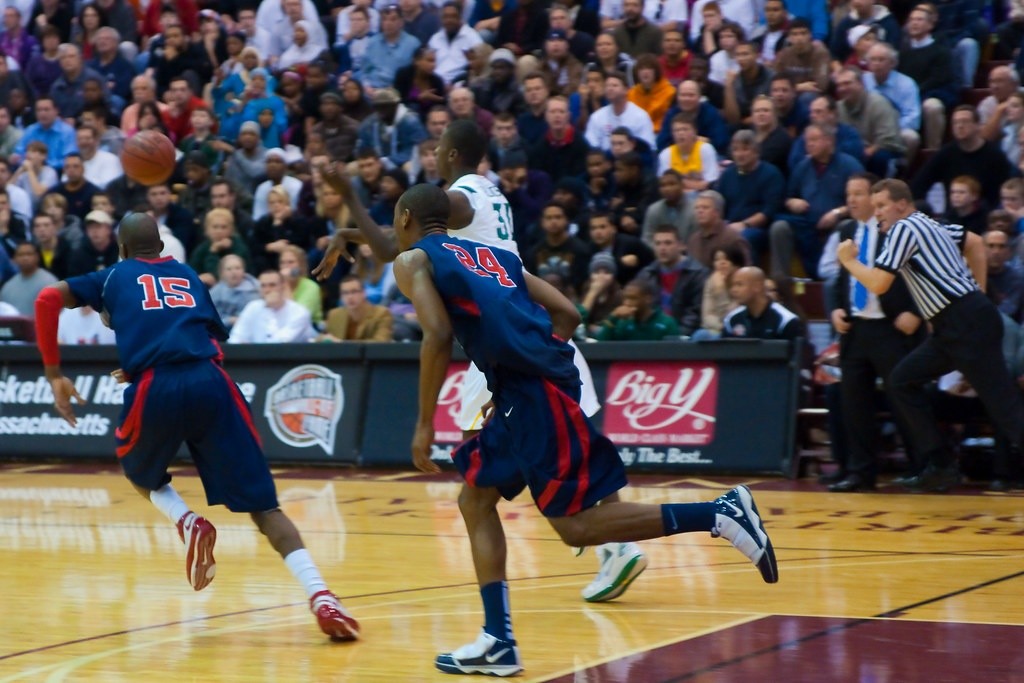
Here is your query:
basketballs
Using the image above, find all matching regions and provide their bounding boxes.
[120,130,178,186]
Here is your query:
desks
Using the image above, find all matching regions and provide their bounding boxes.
[0,342,367,466]
[359,341,806,477]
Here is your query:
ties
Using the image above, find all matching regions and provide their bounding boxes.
[855,223,868,308]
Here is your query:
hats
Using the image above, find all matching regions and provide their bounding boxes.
[849,24,879,45]
[84,211,115,229]
[197,10,220,24]
[546,30,565,43]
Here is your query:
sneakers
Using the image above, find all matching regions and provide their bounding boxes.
[177,514,216,589]
[580,543,648,601]
[435,631,522,677]
[712,483,779,585]
[310,593,360,641]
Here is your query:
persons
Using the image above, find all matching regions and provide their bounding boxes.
[393,184,780,678]
[433,118,648,604]
[0,0,1024,493]
[35,214,364,640]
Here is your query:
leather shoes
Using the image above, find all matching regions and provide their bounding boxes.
[902,463,961,493]
[828,465,875,492]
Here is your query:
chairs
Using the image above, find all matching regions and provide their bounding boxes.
[796,394,1006,484]
